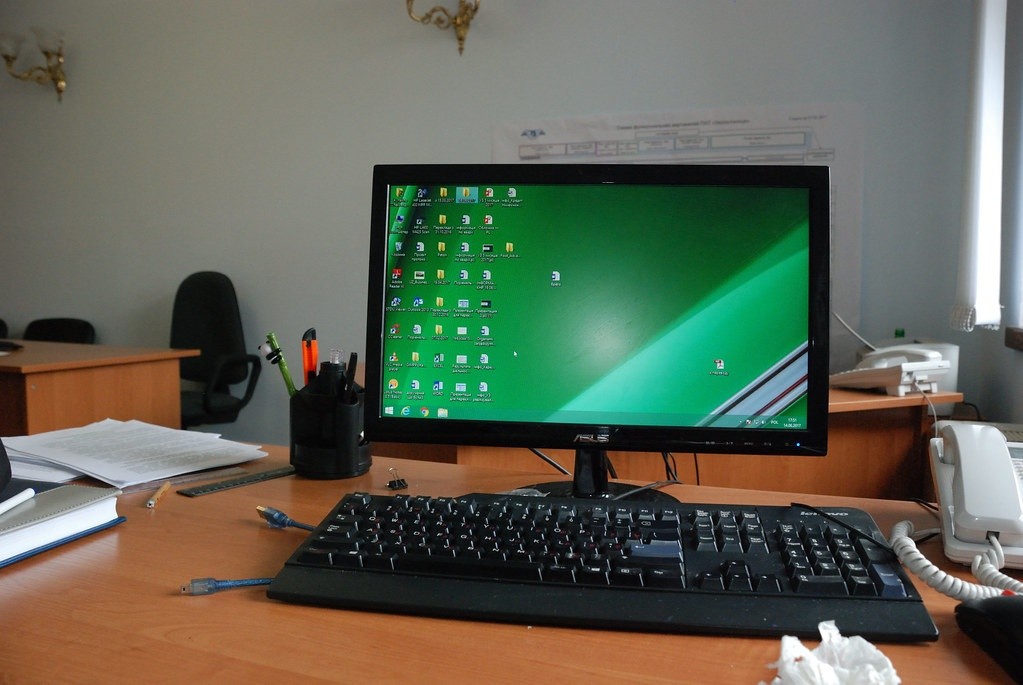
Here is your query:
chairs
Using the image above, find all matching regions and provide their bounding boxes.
[23,318,96,346]
[167,271,261,432]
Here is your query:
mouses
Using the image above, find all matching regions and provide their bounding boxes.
[956,588,1023,685]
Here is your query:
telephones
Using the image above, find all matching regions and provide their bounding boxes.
[831,348,952,398]
[926,421,1023,570]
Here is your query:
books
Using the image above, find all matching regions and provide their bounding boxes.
[0,478,125,567]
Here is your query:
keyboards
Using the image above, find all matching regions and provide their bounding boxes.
[266,491,940,643]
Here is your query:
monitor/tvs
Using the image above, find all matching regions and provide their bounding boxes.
[363,164,829,504]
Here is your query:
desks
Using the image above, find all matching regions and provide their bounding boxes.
[0,337,202,431]
[347,361,964,505]
[0,444,1023,685]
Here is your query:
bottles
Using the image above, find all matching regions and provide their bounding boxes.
[289,362,372,478]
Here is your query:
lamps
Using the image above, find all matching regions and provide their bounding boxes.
[407,0,484,57]
[0,25,69,105]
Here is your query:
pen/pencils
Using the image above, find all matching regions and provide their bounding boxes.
[146,480,171,508]
[258,326,358,402]
